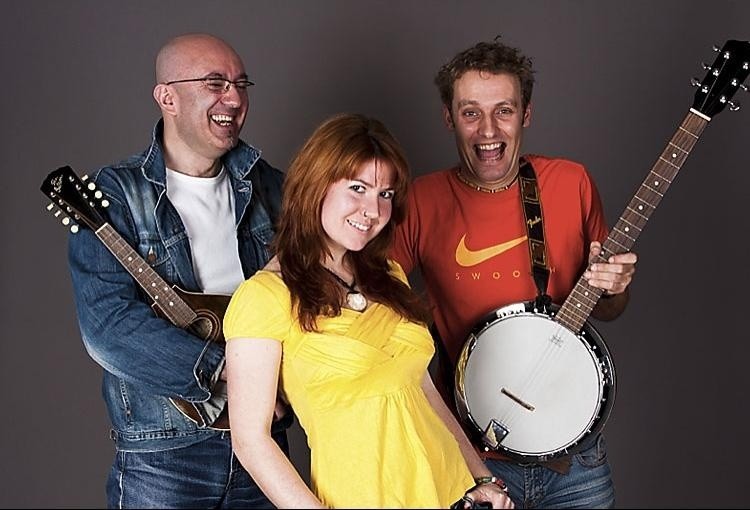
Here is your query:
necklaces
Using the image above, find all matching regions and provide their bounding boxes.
[456,163,519,193]
[322,262,368,312]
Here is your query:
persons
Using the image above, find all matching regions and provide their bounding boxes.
[380,42,639,509]
[222,115,516,510]
[67,33,294,509]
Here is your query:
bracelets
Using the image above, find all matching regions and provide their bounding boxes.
[476,475,511,494]
[599,294,616,301]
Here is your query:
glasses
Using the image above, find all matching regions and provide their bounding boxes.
[166,78,255,91]
